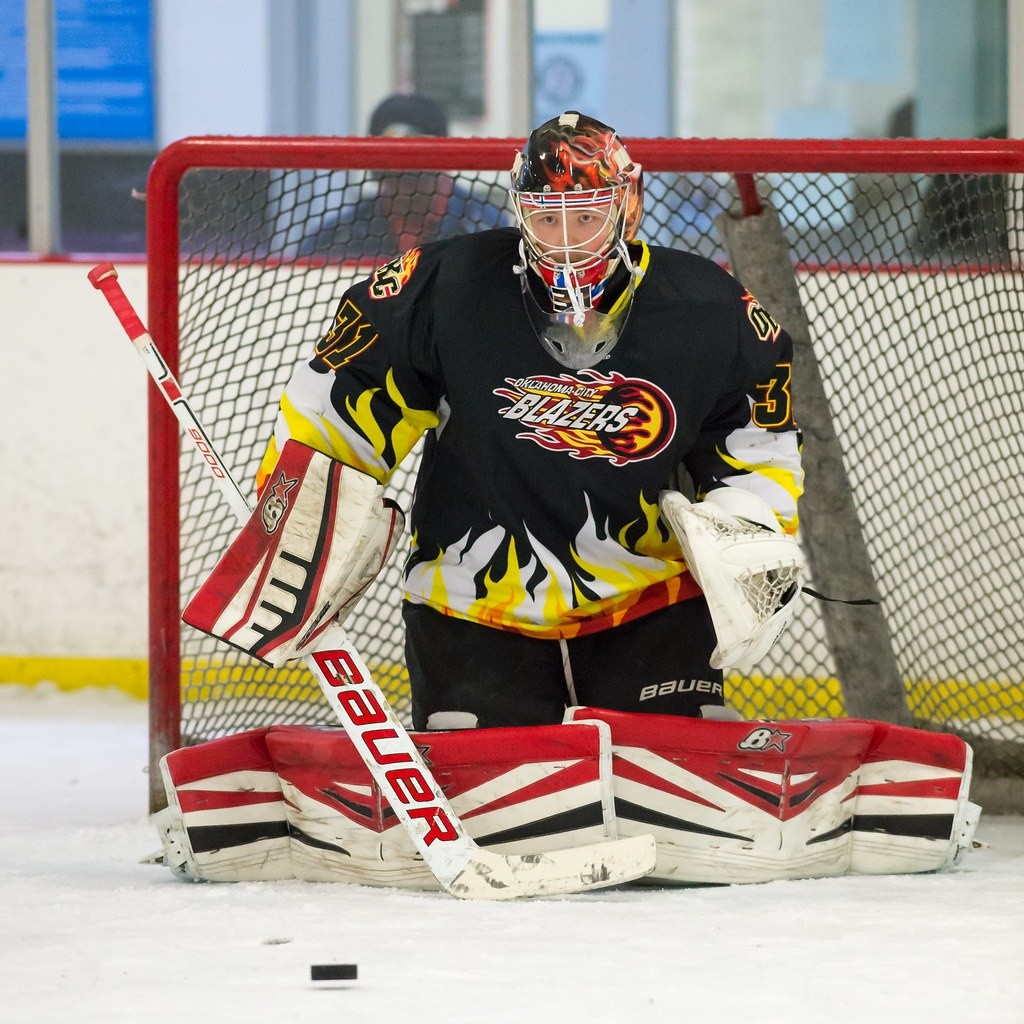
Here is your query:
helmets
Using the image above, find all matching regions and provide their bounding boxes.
[512,110,647,312]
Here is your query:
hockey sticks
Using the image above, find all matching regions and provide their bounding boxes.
[86,261,658,901]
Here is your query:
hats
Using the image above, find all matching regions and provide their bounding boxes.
[368,92,448,137]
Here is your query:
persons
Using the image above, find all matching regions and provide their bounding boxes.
[258,109,808,889]
[296,95,508,265]
[849,100,914,230]
